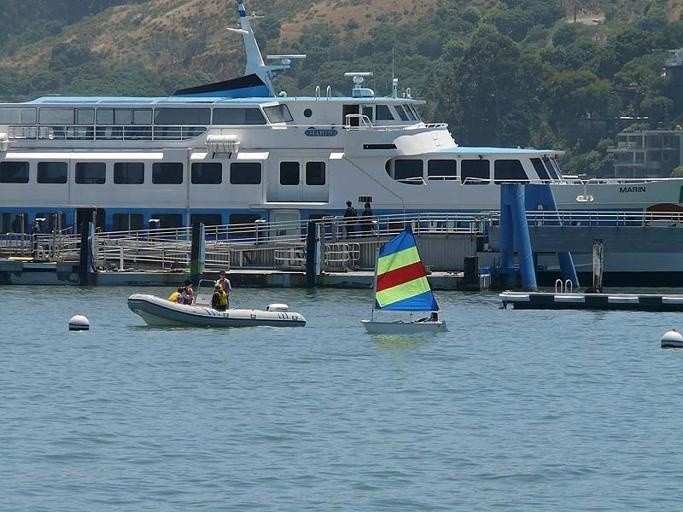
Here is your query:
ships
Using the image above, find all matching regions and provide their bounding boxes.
[0,0,683,269]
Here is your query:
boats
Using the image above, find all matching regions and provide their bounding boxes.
[125,294,306,327]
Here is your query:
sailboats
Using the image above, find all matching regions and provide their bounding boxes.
[360,223,449,334]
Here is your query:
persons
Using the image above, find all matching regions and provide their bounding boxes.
[217,271,231,310]
[32,220,41,235]
[343,200,357,237]
[213,283,230,312]
[361,202,375,235]
[184,279,195,305]
[168,287,183,303]
[12,215,20,234]
